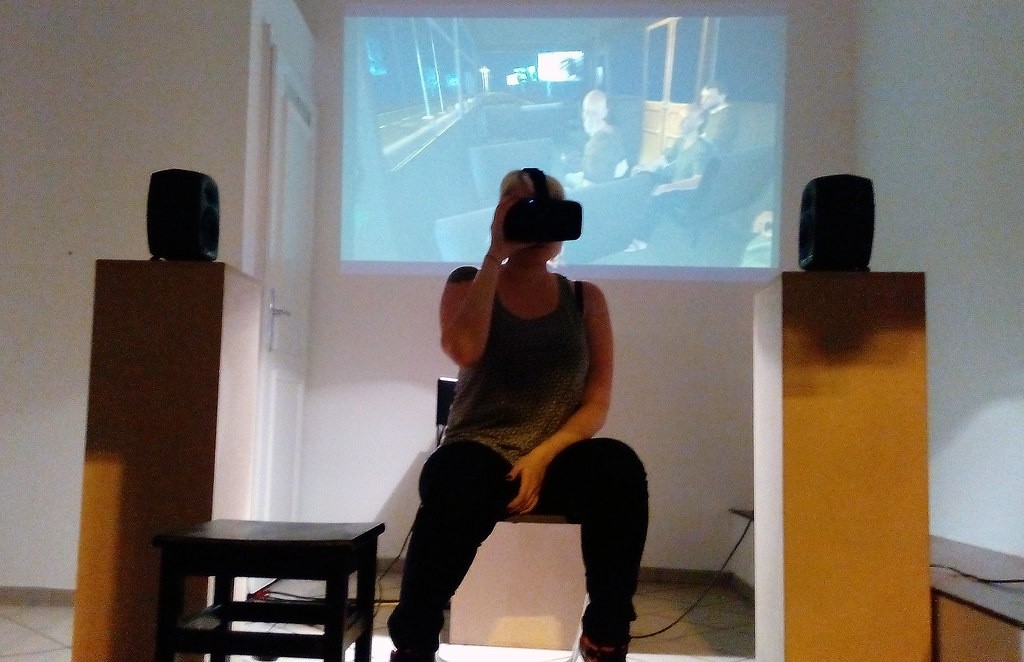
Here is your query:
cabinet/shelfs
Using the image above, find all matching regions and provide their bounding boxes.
[71,258,263,662]
[751,270,934,662]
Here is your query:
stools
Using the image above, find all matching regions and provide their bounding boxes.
[151,518,387,662]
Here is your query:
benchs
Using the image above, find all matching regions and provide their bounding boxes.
[729,508,1024,662]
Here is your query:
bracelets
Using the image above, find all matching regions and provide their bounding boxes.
[487,254,501,266]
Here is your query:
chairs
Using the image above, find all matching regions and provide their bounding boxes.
[437,376,586,649]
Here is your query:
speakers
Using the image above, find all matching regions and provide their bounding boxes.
[146,168,220,260]
[799,173,875,274]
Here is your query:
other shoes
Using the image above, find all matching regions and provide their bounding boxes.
[579,634,631,662]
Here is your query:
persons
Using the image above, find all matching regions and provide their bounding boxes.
[388,169,649,662]
